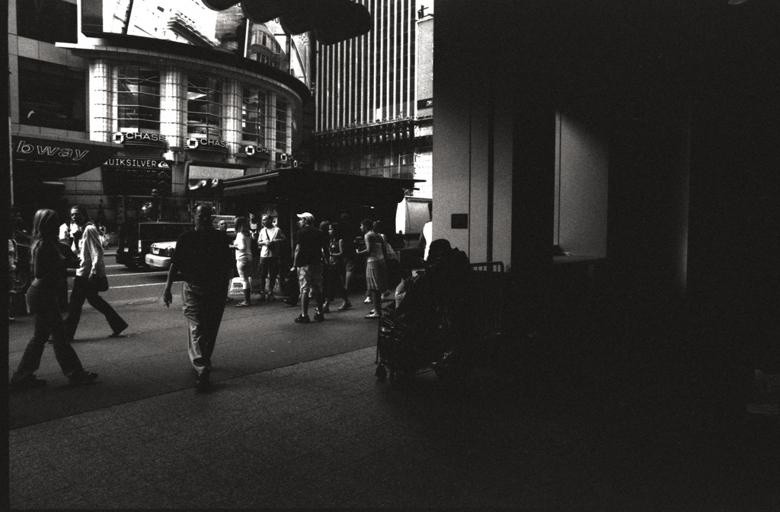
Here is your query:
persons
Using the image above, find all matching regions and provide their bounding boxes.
[217,219,239,305]
[394,239,450,309]
[8,205,128,387]
[228,208,400,323]
[162,206,232,393]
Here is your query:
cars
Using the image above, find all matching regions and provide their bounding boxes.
[145,241,178,270]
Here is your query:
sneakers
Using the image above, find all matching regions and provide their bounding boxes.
[338,302,351,310]
[314,312,324,322]
[295,314,310,323]
[315,304,329,312]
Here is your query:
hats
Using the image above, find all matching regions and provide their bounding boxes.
[297,212,315,223]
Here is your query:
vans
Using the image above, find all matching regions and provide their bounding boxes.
[115,223,197,269]
